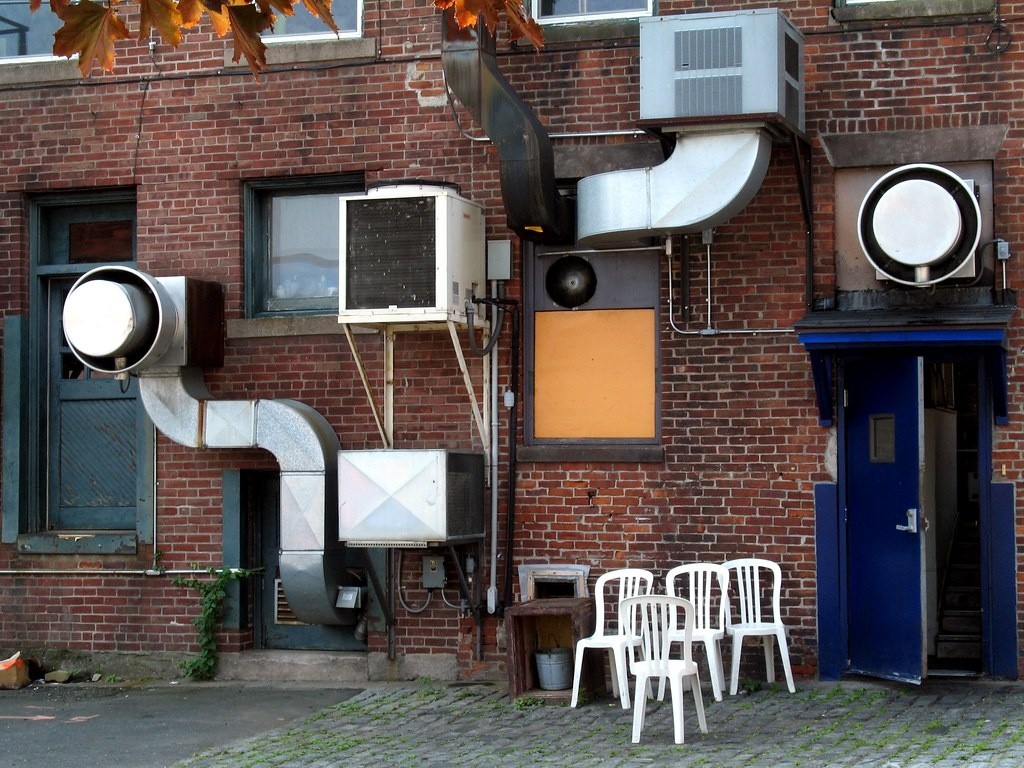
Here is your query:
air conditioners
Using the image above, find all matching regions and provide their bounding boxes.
[338,176,488,333]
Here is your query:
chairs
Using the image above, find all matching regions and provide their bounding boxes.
[570,557,796,745]
